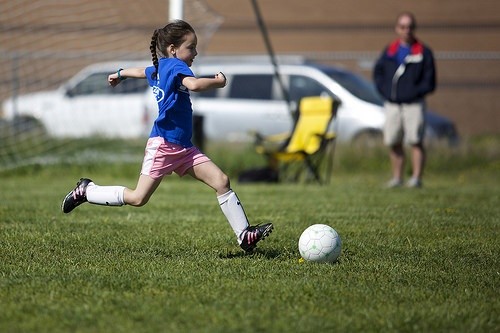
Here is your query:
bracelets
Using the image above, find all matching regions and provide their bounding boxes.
[117,68,127,80]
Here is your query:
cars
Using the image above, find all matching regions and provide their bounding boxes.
[0,61,150,137]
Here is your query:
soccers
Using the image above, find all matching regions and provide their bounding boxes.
[298,224,342,262]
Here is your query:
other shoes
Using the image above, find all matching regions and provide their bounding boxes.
[405,177,424,189]
[386,178,403,188]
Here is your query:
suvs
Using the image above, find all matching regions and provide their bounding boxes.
[142,56,457,148]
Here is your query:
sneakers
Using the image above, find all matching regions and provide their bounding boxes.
[238,221,275,253]
[61,177,93,214]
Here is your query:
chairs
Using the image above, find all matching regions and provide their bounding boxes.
[247,95,337,186]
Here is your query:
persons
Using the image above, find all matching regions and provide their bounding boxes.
[374,12,435,188]
[62,20,274,252]
